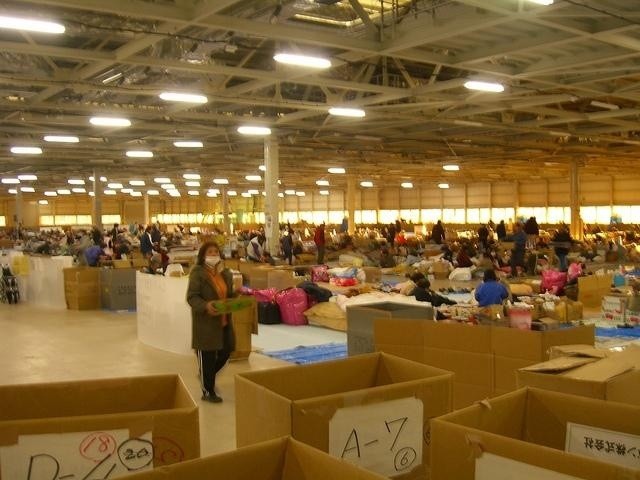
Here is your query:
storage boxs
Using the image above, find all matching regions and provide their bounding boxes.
[426,383,638,480]
[231,348,457,457]
[1,243,638,364]
[100,434,397,480]
[0,371,203,480]
[512,343,638,401]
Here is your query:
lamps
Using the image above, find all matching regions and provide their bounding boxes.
[530,0,553,6]
[463,77,505,94]
[0,15,66,35]
[273,53,332,70]
[159,93,207,103]
[90,117,131,128]
[329,107,365,119]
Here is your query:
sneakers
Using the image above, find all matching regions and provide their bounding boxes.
[201,394,222,402]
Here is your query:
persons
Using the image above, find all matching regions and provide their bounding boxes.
[85,220,170,275]
[342,216,348,233]
[380,217,640,278]
[314,224,326,264]
[475,267,509,307]
[410,271,457,322]
[187,240,235,403]
[238,217,303,265]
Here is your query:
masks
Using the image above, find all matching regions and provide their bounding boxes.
[204,255,221,267]
